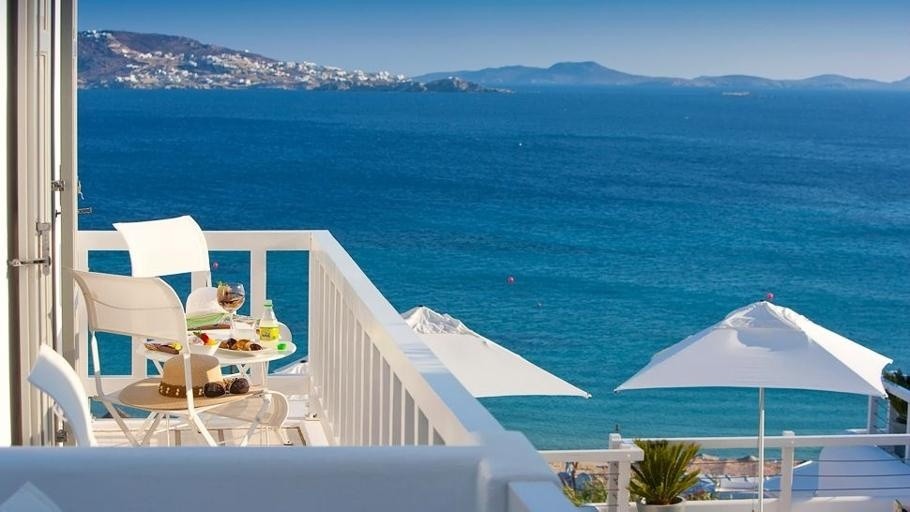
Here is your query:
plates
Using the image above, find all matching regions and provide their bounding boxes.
[216,340,269,356]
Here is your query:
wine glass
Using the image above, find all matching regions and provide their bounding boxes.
[217,283,245,335]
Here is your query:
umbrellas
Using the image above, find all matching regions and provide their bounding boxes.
[613,298,896,511]
[268,305,594,403]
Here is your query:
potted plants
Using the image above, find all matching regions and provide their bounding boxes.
[877,369,909,459]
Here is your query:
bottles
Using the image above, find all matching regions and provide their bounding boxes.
[259,300,279,346]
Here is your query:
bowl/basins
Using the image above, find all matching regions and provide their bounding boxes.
[189,340,219,356]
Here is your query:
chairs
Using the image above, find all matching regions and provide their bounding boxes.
[68,268,292,447]
[112,216,264,384]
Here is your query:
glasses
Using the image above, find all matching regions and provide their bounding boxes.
[203,377,252,399]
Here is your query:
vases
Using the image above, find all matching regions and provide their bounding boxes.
[627,438,698,512]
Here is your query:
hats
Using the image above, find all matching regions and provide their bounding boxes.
[118,353,263,412]
